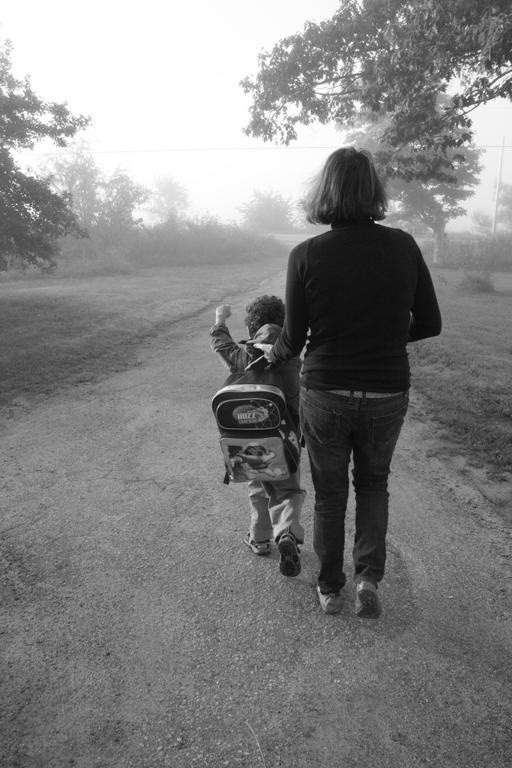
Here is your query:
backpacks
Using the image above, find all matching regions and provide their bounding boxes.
[212,347,304,484]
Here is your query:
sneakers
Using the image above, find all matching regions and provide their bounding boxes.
[355,582,382,619]
[318,585,341,614]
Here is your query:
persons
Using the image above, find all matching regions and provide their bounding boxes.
[208,295,306,577]
[251,147,441,619]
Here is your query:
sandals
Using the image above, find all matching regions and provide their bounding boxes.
[243,531,272,554]
[278,533,302,576]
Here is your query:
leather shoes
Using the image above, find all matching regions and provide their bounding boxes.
[323,389,408,397]
